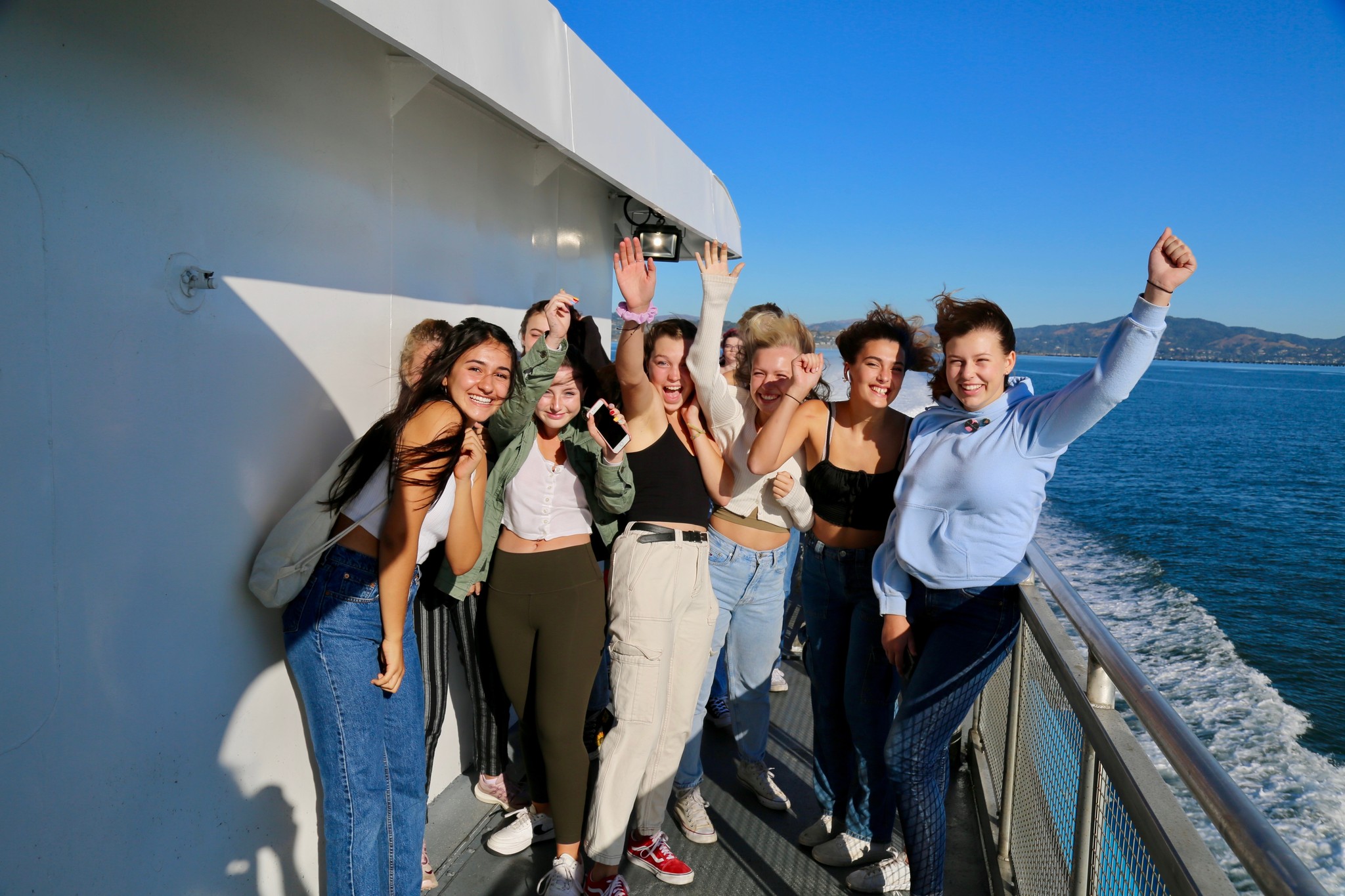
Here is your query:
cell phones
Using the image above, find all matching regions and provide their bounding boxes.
[586,398,632,454]
[902,644,914,679]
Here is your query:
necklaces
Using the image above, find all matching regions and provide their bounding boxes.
[851,414,881,422]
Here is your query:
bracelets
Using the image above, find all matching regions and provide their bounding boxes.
[615,301,657,335]
[1146,279,1173,294]
[687,423,706,440]
[785,393,801,404]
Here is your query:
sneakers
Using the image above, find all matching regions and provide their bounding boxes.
[474,770,532,814]
[673,785,717,843]
[846,846,911,893]
[537,850,585,896]
[736,759,790,809]
[799,814,847,847]
[421,838,438,891]
[704,695,732,728]
[583,705,618,760]
[486,804,556,857]
[626,828,695,885]
[583,867,630,896]
[812,832,892,867]
[769,668,788,692]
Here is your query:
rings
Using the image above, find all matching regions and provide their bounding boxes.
[635,259,644,262]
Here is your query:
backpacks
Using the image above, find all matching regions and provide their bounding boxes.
[249,399,453,608]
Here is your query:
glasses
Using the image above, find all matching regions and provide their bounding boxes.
[724,344,744,351]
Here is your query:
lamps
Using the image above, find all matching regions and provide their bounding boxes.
[630,210,684,267]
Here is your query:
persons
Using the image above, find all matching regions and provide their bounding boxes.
[277,316,526,896]
[746,302,938,865]
[399,234,831,896]
[868,226,1196,896]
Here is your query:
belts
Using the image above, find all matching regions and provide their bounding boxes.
[630,522,707,544]
